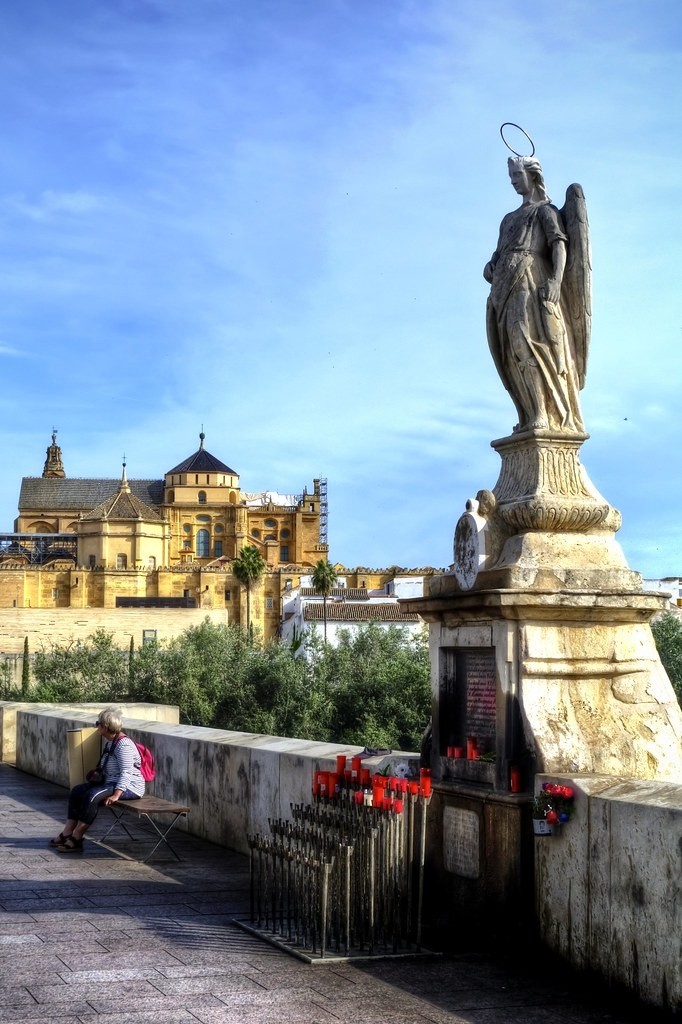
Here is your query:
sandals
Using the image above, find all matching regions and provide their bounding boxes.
[56,836,85,853]
[50,832,72,846]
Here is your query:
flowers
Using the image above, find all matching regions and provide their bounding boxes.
[531,781,575,825]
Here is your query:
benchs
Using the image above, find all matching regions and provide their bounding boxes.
[95,793,189,866]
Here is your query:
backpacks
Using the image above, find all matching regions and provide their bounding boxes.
[112,735,156,782]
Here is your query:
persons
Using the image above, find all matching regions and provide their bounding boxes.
[50,708,145,852]
[484,122,592,435]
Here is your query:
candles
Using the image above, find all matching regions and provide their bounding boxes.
[363,792,373,806]
[408,781,418,794]
[336,756,346,775]
[419,767,432,796]
[380,774,388,789]
[444,734,480,761]
[371,773,380,786]
[382,797,392,810]
[328,772,339,796]
[510,766,520,793]
[373,785,385,808]
[344,769,352,782]
[320,774,329,795]
[352,756,362,782]
[312,770,331,793]
[392,799,403,814]
[359,768,370,785]
[388,776,397,790]
[398,779,408,792]
[354,791,363,804]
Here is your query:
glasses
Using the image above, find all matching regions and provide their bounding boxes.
[95,721,103,727]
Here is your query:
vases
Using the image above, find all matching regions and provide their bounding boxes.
[531,812,553,837]
